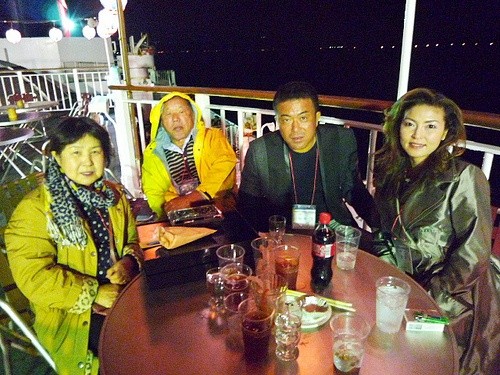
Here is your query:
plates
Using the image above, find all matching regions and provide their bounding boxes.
[280,291,332,328]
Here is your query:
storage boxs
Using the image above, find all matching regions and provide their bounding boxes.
[137,211,259,290]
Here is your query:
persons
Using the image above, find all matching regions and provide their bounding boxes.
[369,87,500,375]
[239,80,382,254]
[4,117,144,375]
[141,90,237,220]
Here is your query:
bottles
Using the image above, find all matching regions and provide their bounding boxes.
[311,212,336,288]
[17,100,24,109]
[8,111,21,121]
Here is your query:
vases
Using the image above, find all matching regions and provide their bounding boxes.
[8,107,18,121]
[17,100,24,108]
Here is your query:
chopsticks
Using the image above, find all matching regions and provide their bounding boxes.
[285,289,356,313]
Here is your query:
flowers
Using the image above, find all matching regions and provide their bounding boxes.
[12,93,33,101]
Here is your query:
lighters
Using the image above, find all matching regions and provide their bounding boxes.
[415,311,449,325]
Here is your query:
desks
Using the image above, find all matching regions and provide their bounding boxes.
[0,128,42,177]
[0,99,58,109]
[0,112,52,139]
[99,234,460,375]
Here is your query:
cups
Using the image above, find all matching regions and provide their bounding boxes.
[268,214,286,245]
[224,292,247,329]
[252,273,289,312]
[205,267,228,309]
[250,237,280,278]
[237,297,276,343]
[335,224,361,270]
[276,294,302,345]
[215,244,246,270]
[219,263,253,293]
[273,246,300,291]
[375,275,410,330]
[329,313,372,371]
[274,315,302,360]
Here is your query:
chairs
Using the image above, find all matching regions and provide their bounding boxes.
[0,175,58,375]
[450,255,500,375]
[31,100,79,174]
